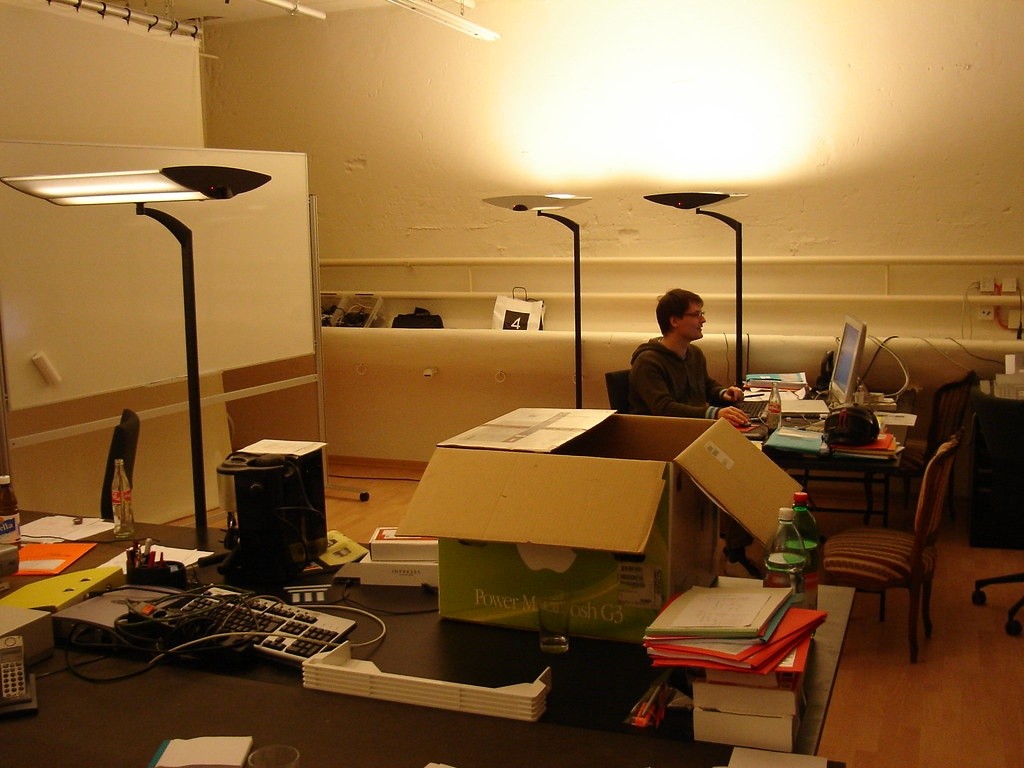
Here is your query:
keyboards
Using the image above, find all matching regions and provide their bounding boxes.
[738,399,769,420]
[180,586,357,670]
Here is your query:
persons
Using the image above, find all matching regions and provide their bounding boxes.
[629,288,762,580]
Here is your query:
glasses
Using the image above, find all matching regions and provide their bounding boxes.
[683,310,706,319]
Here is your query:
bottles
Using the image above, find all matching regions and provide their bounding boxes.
[763,508,807,615]
[786,492,819,613]
[111,460,135,538]
[0,475,21,550]
[767,382,781,436]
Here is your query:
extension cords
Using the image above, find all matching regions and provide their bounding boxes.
[128,602,179,635]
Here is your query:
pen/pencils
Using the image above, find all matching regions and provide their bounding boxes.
[744,393,764,397]
[641,682,664,717]
[877,414,904,418]
[126,537,163,568]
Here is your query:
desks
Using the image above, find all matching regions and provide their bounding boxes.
[968,387,1024,550]
[0,505,733,768]
[762,385,917,529]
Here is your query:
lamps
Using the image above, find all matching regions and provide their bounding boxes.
[644,191,748,390]
[482,192,592,408]
[0,158,273,553]
[388,1,501,44]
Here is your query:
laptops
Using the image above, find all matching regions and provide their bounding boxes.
[781,399,830,418]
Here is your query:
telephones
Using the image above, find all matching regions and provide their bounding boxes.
[0,635,39,719]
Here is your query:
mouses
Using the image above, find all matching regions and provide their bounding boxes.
[741,421,752,427]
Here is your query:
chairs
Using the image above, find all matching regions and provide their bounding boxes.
[818,428,963,665]
[860,368,975,529]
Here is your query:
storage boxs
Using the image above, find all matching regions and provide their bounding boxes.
[396,406,804,645]
[367,525,438,561]
[334,545,439,590]
[689,666,828,768]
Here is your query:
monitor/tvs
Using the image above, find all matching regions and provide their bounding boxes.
[830,314,866,407]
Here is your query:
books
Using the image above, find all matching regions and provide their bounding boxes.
[693,667,806,754]
[764,427,905,460]
[748,373,809,390]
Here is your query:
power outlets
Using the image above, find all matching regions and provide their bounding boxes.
[979,277,995,292]
[1002,278,1017,292]
[1007,310,1024,331]
[979,305,995,321]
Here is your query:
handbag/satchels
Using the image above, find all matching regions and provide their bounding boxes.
[392,307,444,329]
[491,287,545,331]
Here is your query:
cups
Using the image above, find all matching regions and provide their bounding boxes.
[537,586,573,653]
